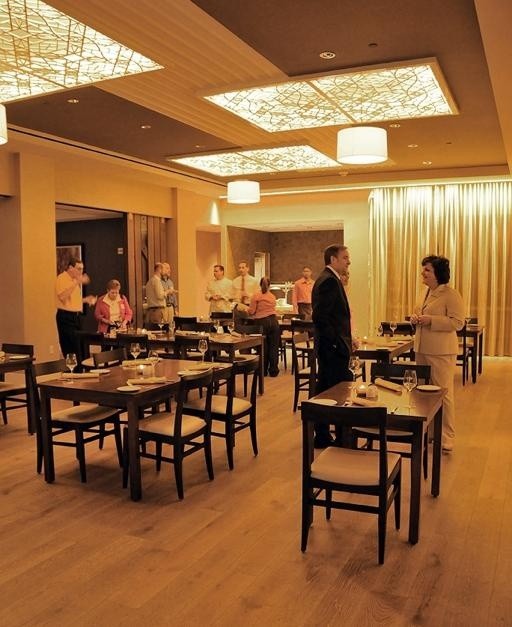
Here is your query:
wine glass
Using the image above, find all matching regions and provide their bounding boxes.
[198,338,209,365]
[402,369,418,408]
[212,319,235,340]
[374,324,383,337]
[147,349,159,383]
[464,317,471,329]
[389,319,397,337]
[347,355,361,389]
[190,310,216,323]
[158,318,165,335]
[65,351,78,375]
[130,342,142,370]
[168,319,176,339]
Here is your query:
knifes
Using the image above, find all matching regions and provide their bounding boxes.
[54,378,78,382]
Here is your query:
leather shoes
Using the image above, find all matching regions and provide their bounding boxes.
[313,437,335,448]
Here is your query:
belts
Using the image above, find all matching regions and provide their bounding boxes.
[149,306,165,310]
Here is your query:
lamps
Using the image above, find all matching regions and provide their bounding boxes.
[0,100,8,147]
[227,142,261,206]
[335,68,388,165]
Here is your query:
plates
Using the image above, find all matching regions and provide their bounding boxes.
[9,354,31,359]
[416,383,442,391]
[88,368,112,374]
[309,397,338,405]
[178,369,202,376]
[116,385,142,392]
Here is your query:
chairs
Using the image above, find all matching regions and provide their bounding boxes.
[2,301,485,563]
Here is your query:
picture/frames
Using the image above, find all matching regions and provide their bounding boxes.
[56,242,86,315]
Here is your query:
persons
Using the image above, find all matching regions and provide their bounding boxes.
[247,277,280,377]
[161,263,179,326]
[144,262,174,330]
[291,266,316,341]
[233,260,259,305]
[203,265,238,318]
[311,243,353,449]
[55,259,97,373]
[410,255,465,456]
[340,270,358,352]
[94,279,132,353]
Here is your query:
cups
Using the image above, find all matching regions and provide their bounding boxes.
[110,321,151,335]
[392,355,411,362]
[375,333,415,349]
[240,331,247,339]
[173,328,210,339]
[275,297,288,305]
[352,328,369,349]
[354,384,378,398]
